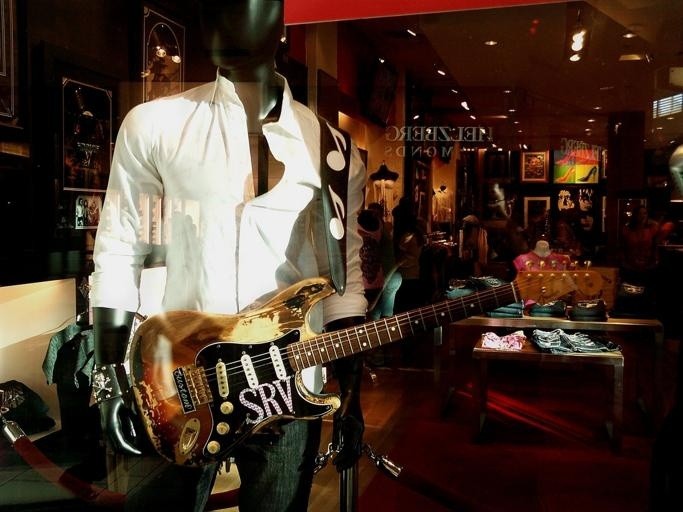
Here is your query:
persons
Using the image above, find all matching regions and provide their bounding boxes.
[76,198,100,226]
[618,206,662,316]
[88,0,369,512]
[369,196,425,366]
[358,210,384,293]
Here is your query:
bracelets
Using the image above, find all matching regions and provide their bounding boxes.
[91,362,131,404]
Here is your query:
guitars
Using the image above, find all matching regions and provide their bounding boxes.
[129,260,602,468]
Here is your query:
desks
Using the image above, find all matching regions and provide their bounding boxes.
[432,310,663,454]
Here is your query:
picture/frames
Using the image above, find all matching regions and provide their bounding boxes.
[519,151,550,184]
[0,0,190,195]
